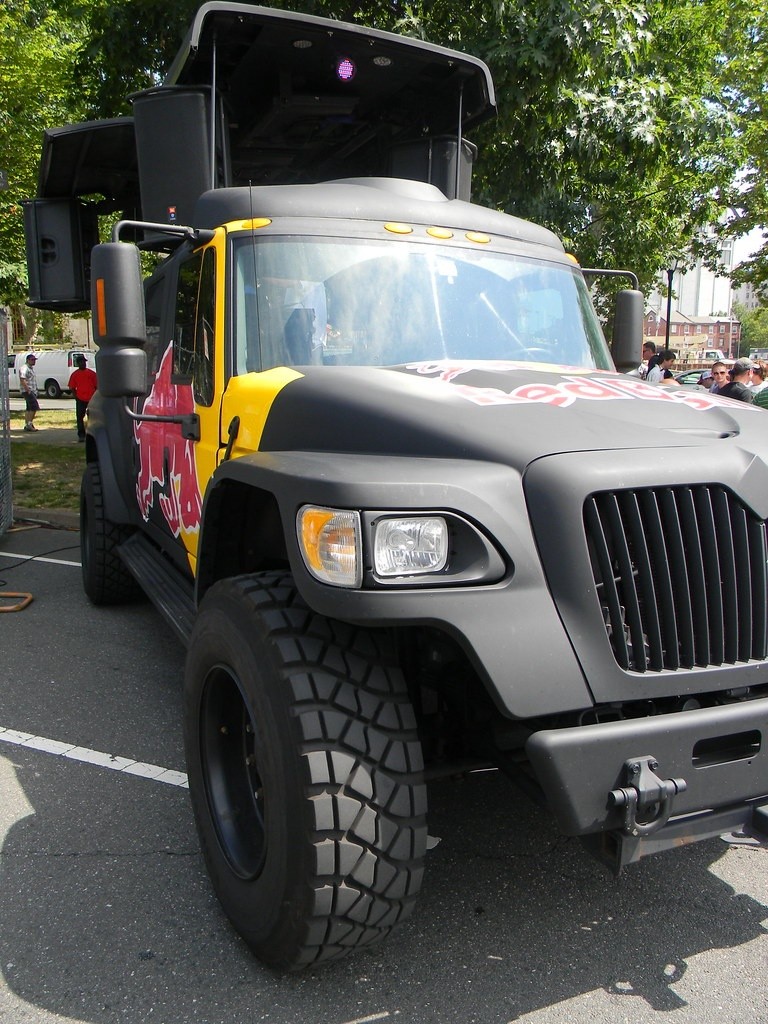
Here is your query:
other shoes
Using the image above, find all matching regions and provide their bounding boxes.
[24,423,38,432]
[78,437,85,442]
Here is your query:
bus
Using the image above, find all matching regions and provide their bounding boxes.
[749,346,768,360]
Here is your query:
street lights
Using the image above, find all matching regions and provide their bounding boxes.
[664,253,679,356]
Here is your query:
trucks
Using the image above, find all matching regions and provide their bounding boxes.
[685,347,737,367]
[6,348,100,399]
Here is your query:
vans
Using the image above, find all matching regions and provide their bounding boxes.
[26,0,767,979]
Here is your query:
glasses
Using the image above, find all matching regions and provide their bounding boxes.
[713,372,725,376]
[642,350,649,354]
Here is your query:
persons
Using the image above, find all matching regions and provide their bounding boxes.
[712,362,730,393]
[68,356,97,442]
[748,360,768,410]
[643,342,674,380]
[647,350,677,381]
[18,355,39,432]
[717,357,760,404]
[697,371,718,392]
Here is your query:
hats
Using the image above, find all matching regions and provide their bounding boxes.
[27,354,37,359]
[697,371,712,384]
[734,357,760,368]
[76,355,88,361]
